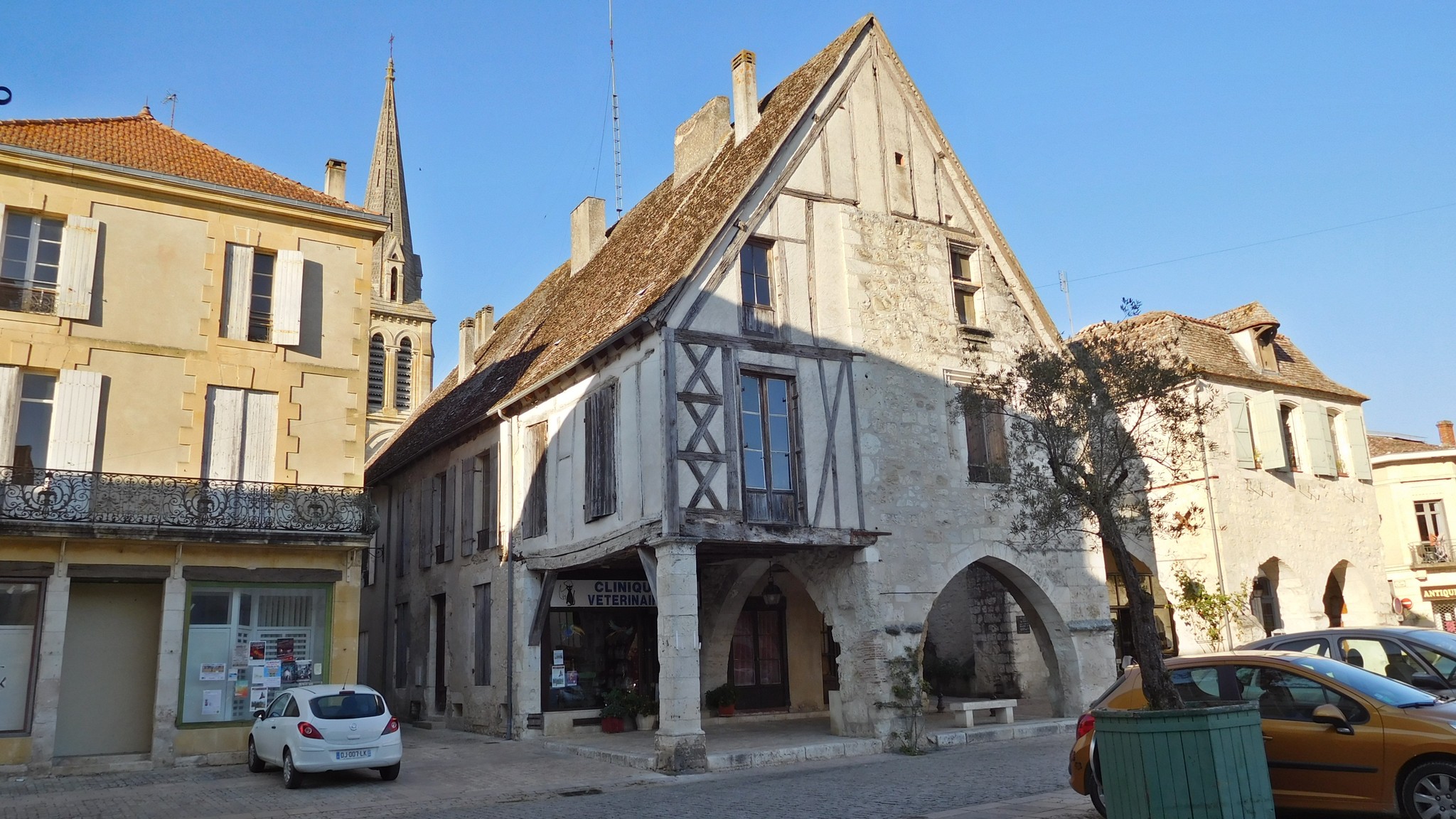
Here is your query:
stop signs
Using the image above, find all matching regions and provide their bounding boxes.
[1400,599,1413,610]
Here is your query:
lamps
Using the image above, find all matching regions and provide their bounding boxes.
[761,561,781,613]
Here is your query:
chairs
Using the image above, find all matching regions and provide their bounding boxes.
[1341,647,1363,668]
[1385,660,1418,686]
[1236,669,1301,720]
[337,698,362,720]
[1416,544,1447,563]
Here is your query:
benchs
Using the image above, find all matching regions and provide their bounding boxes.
[951,699,1017,728]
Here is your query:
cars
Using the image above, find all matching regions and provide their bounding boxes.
[1066,649,1456,819]
[247,684,403,787]
[1195,626,1455,708]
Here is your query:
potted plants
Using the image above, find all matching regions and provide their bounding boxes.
[599,688,626,732]
[705,684,739,717]
[622,691,659,730]
